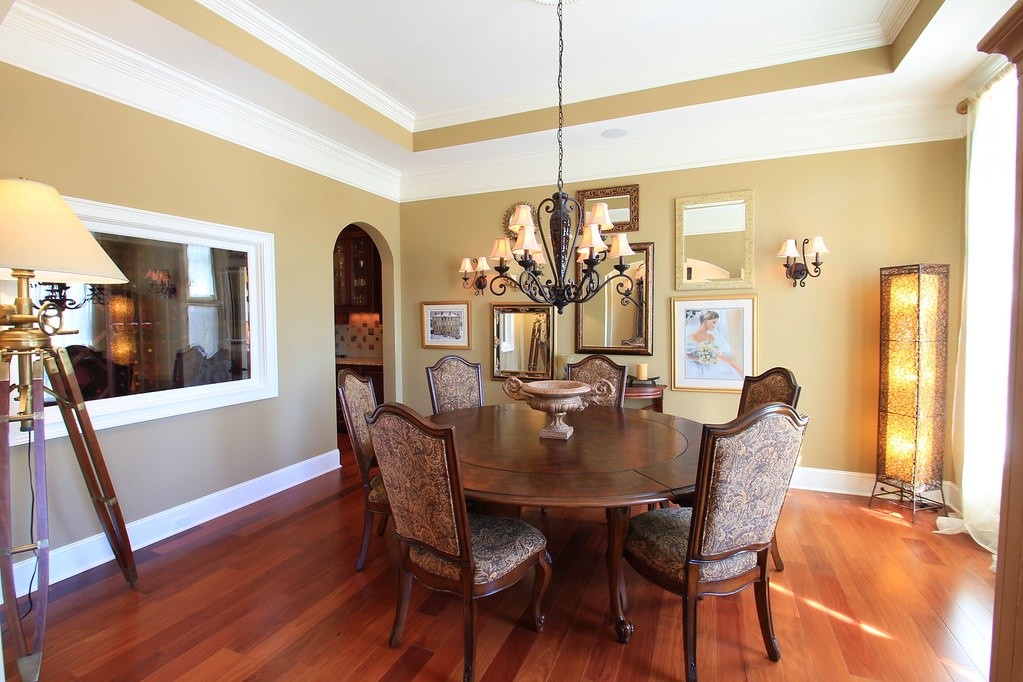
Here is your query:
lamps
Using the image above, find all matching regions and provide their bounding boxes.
[0,177,132,434]
[487,0,636,314]
[777,236,831,288]
[458,256,491,295]
[621,263,646,310]
[868,263,951,524]
[107,289,137,397]
[144,268,177,301]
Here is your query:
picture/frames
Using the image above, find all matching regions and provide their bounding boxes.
[420,301,473,351]
[668,294,759,394]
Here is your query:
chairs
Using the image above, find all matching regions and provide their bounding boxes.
[337,368,392,574]
[425,355,484,414]
[66,344,108,400]
[603,401,810,682]
[363,402,556,682]
[193,348,239,387]
[566,355,629,408]
[659,367,803,574]
[170,344,209,387]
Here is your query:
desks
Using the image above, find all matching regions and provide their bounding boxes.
[422,403,707,646]
[624,383,667,414]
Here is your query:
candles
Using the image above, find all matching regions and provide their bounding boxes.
[637,363,648,381]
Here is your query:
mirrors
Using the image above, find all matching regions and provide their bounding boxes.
[490,301,557,384]
[576,183,640,232]
[574,242,655,356]
[674,190,755,291]
[503,200,542,243]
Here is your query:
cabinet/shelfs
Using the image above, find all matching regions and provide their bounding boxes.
[334,229,382,325]
[336,364,383,435]
